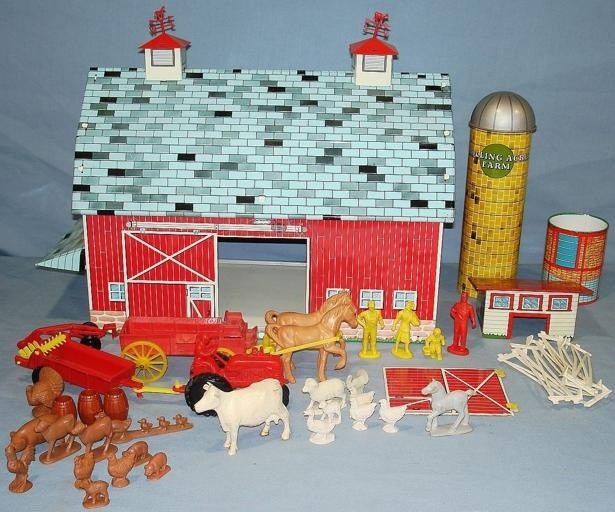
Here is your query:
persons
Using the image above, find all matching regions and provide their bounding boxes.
[426,328,445,360]
[356,302,385,354]
[392,302,420,352]
[450,293,475,345]
